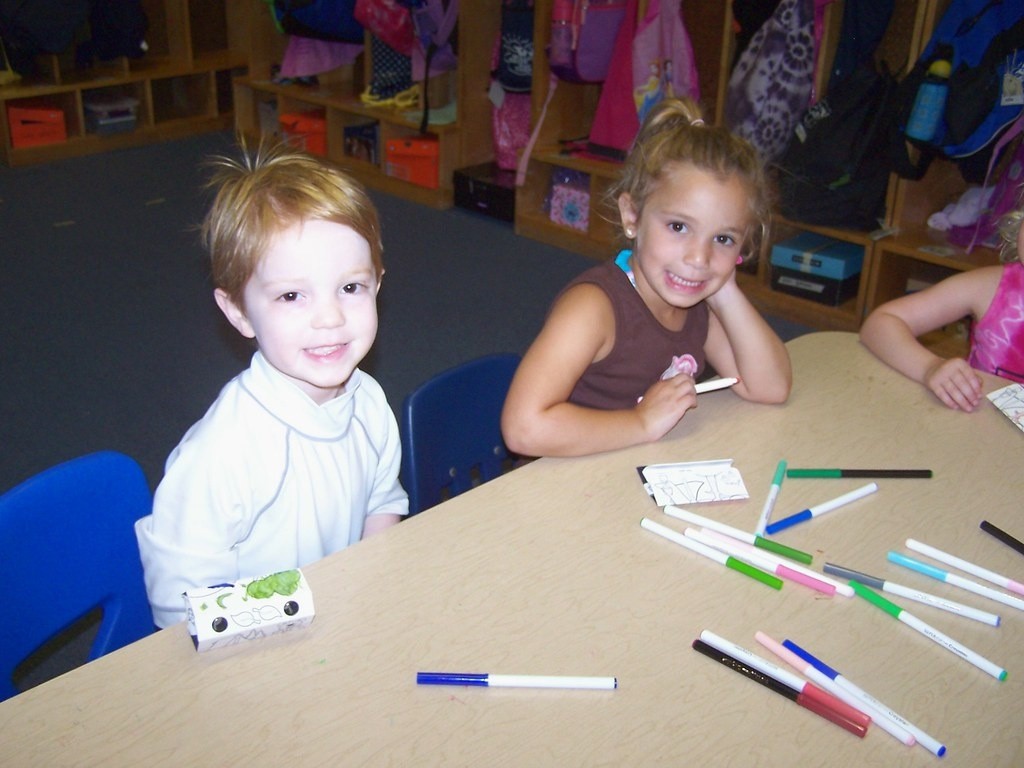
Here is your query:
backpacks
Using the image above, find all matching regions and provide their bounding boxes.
[776,57,908,231]
[871,0,1024,182]
[965,110,1024,255]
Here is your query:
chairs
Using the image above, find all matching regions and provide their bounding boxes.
[400,350,528,520]
[0,449,161,703]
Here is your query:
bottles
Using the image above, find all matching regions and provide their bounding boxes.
[904,60,951,140]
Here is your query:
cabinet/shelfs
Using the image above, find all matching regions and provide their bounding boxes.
[0,0,1024,364]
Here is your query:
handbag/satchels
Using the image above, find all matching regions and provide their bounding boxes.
[262,0,626,170]
[721,0,825,175]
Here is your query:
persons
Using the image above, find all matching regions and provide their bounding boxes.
[134,152,409,630]
[859,209,1024,412]
[501,94,792,456]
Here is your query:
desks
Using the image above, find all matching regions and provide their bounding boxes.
[0,328,1024,768]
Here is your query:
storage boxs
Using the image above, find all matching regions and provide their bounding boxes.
[150,78,208,119]
[278,106,327,158]
[768,230,864,309]
[217,68,249,112]
[382,134,440,190]
[342,121,377,164]
[548,181,594,234]
[182,567,316,652]
[82,90,140,137]
[251,94,277,129]
[451,157,516,221]
[6,101,71,150]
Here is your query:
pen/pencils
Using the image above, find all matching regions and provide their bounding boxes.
[886,552,1024,611]
[847,580,1007,681]
[822,563,1001,627]
[764,482,878,535]
[786,469,932,478]
[753,459,787,537]
[699,529,855,597]
[700,629,872,727]
[636,377,739,404]
[683,527,836,596]
[904,538,1024,595]
[663,505,813,564]
[979,520,1024,556]
[416,672,617,690]
[781,640,946,757]
[692,640,868,739]
[640,518,784,591]
[754,631,916,747]
